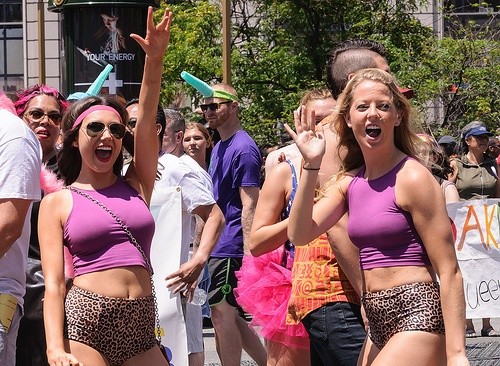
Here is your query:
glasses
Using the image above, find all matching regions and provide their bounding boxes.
[496,145,500,148]
[199,101,232,112]
[86,121,126,139]
[128,120,136,128]
[29,111,62,126]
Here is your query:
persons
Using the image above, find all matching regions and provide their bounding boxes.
[416,121,500,338]
[120,83,267,366]
[286,40,390,366]
[37,6,173,366]
[234,89,338,366]
[284,68,471,366]
[0,85,70,366]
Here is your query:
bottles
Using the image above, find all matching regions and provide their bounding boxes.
[181,288,207,306]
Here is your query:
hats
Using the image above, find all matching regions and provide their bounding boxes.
[465,126,494,137]
[437,136,456,144]
